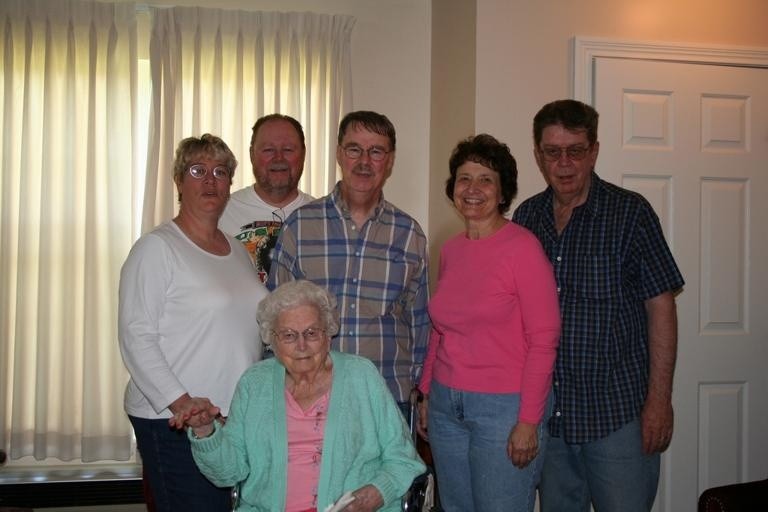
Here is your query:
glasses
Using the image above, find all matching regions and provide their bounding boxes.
[272,327,326,343]
[339,143,392,160]
[537,144,592,162]
[183,165,231,178]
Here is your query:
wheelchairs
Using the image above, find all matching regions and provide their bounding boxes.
[229,388,434,511]
